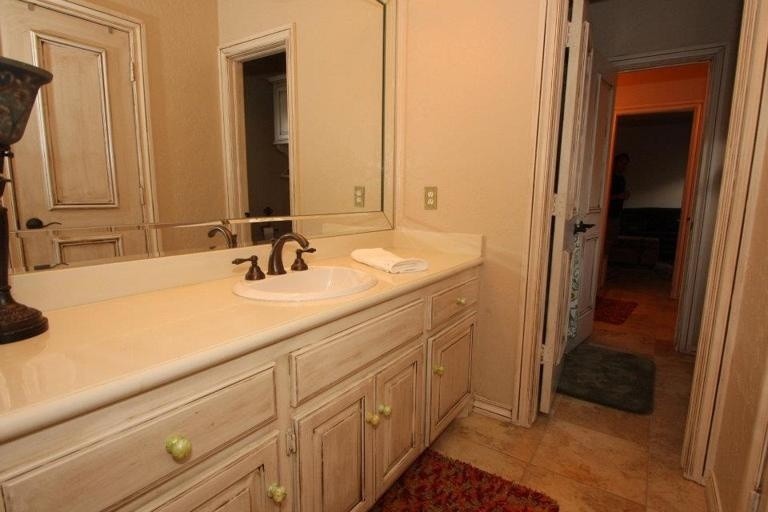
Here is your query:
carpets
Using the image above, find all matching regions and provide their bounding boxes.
[594,297,639,326]
[369,444,561,511]
[551,340,658,415]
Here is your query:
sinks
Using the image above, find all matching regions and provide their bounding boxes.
[231,264,378,301]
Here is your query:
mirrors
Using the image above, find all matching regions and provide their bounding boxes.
[1,0,397,280]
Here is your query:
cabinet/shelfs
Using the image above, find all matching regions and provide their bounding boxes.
[1,336,288,512]
[290,283,427,512]
[428,264,482,446]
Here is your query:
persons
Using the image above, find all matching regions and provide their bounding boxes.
[603,150,630,254]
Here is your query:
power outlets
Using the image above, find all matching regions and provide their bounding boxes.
[354,186,365,207]
[425,186,437,210]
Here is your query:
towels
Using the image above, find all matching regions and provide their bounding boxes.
[350,246,427,276]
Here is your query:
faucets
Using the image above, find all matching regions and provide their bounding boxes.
[207,226,236,249]
[267,232,310,273]
